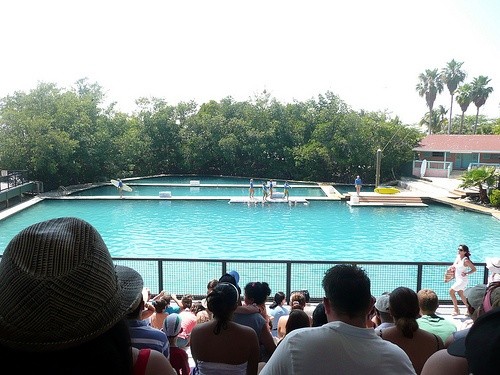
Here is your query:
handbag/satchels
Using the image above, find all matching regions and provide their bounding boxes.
[444,269,455,283]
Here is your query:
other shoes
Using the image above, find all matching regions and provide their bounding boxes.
[452,309,460,315]
[464,311,469,315]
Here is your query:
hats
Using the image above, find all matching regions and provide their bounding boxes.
[163,313,181,336]
[374,295,390,312]
[0,217,143,354]
[483,280,500,310]
[486,257,500,273]
[202,298,209,308]
[464,283,487,308]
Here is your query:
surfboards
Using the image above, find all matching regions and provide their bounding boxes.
[110,179,133,193]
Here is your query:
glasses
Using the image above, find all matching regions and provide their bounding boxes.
[458,248,465,252]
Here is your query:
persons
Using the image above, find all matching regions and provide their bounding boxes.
[268,179,273,200]
[0,258,500,375]
[448,244,477,317]
[282,181,291,201]
[355,176,362,197]
[262,182,269,202]
[117,178,123,199]
[249,179,255,200]
[0,218,146,375]
